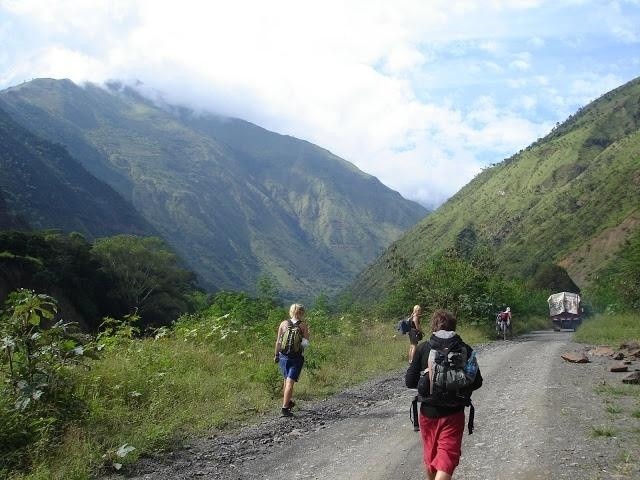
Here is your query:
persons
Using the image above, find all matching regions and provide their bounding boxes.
[495,307,512,340]
[274,303,309,413]
[406,308,483,479]
[408,304,422,364]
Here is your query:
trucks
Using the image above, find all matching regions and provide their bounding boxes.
[547,292,584,332]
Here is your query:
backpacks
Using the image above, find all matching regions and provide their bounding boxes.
[397,316,415,336]
[500,312,508,322]
[409,342,475,435]
[279,320,303,359]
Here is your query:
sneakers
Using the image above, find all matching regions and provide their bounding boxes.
[288,400,295,408]
[281,407,293,417]
